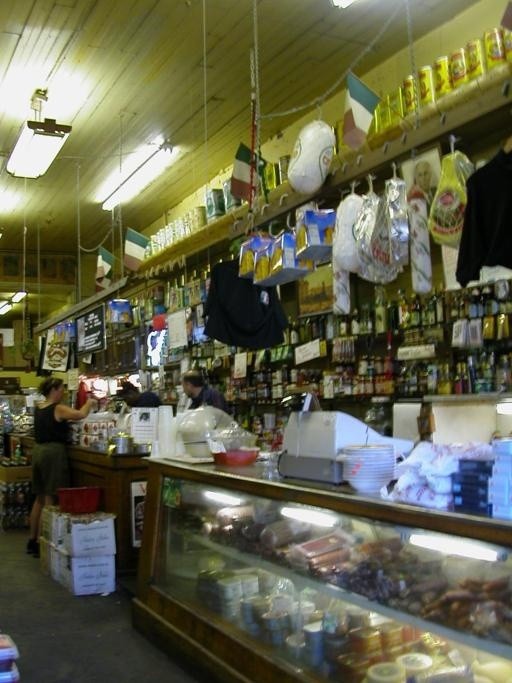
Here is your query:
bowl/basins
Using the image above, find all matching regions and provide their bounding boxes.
[342,444,396,494]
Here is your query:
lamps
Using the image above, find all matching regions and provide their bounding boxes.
[5,88,73,180]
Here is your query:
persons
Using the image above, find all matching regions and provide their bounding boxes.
[407,159,441,217]
[110,380,162,409]
[181,369,231,417]
[25,376,99,559]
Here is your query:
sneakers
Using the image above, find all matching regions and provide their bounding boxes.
[28,539,36,552]
[33,542,40,557]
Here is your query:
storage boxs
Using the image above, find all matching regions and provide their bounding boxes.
[66,511,116,556]
[57,546,117,596]
[53,512,67,544]
[39,536,50,575]
[41,505,60,541]
[49,542,59,580]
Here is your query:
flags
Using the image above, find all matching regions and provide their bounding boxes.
[92,248,114,288]
[122,229,151,274]
[342,74,385,151]
[230,145,267,206]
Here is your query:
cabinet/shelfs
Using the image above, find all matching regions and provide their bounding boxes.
[31,276,129,335]
[9,423,151,567]
[132,456,511,683]
[134,65,511,410]
